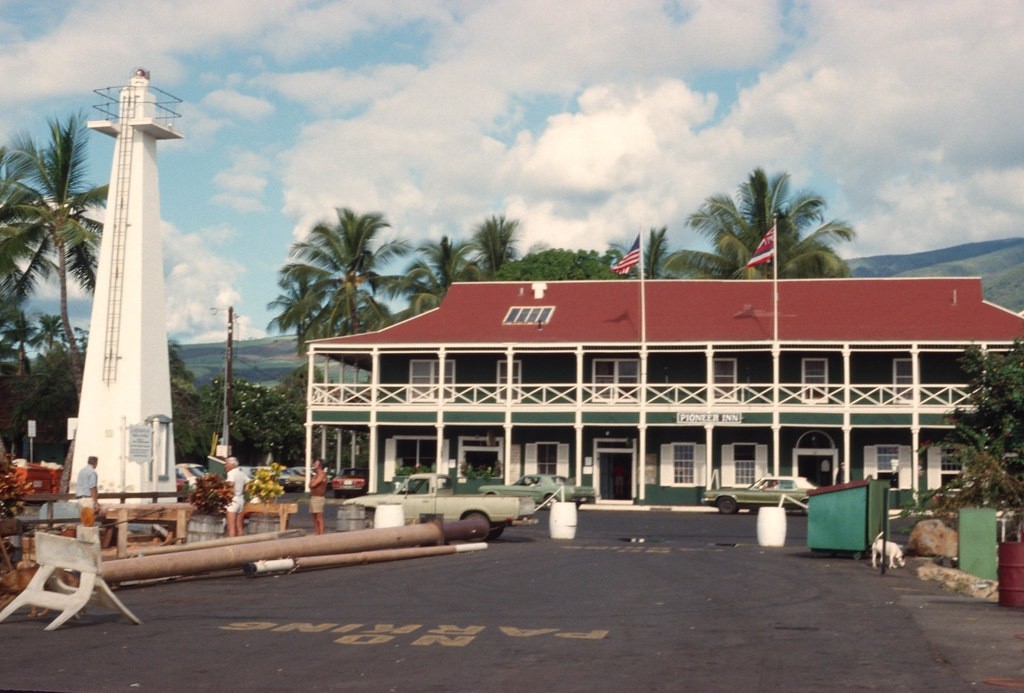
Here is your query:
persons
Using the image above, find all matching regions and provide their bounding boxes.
[838,462,844,483]
[77,456,99,512]
[763,481,778,490]
[224,457,249,537]
[309,458,327,536]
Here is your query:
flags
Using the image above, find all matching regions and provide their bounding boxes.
[610,234,640,274]
[746,226,774,268]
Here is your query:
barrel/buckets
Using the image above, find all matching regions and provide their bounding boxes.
[335,504,365,532]
[248,513,280,535]
[373,502,404,529]
[185,514,226,545]
[997,542,1024,608]
[757,506,787,548]
[549,501,578,541]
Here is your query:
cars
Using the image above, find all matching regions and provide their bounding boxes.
[176,463,210,489]
[241,464,333,492]
[332,466,371,497]
[701,475,822,517]
[175,468,190,496]
[477,473,596,510]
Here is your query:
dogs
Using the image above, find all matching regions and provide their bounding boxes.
[872,531,906,569]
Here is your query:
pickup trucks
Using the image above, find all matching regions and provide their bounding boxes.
[341,473,538,544]
